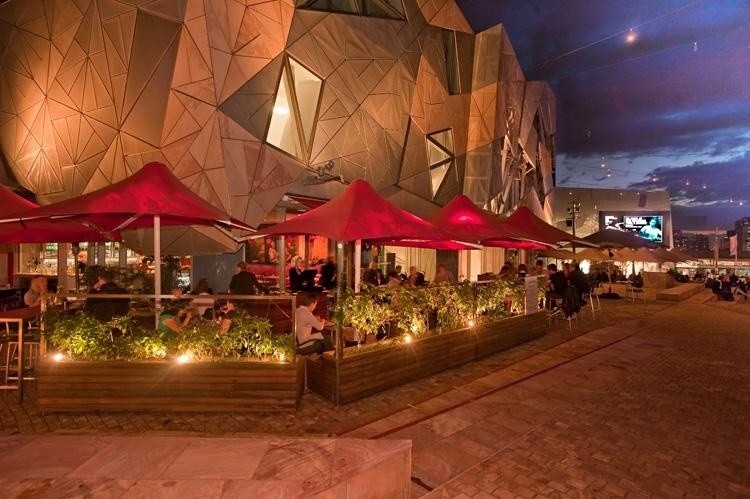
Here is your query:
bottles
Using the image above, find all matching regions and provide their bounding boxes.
[27,257,57,274]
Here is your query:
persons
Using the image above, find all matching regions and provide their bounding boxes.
[703,268,750,301]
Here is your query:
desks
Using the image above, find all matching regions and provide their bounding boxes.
[323,319,336,351]
[0,283,85,401]
[591,280,638,298]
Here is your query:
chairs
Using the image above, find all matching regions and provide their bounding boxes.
[547,297,578,332]
[584,281,602,319]
[295,335,332,395]
[616,281,631,286]
